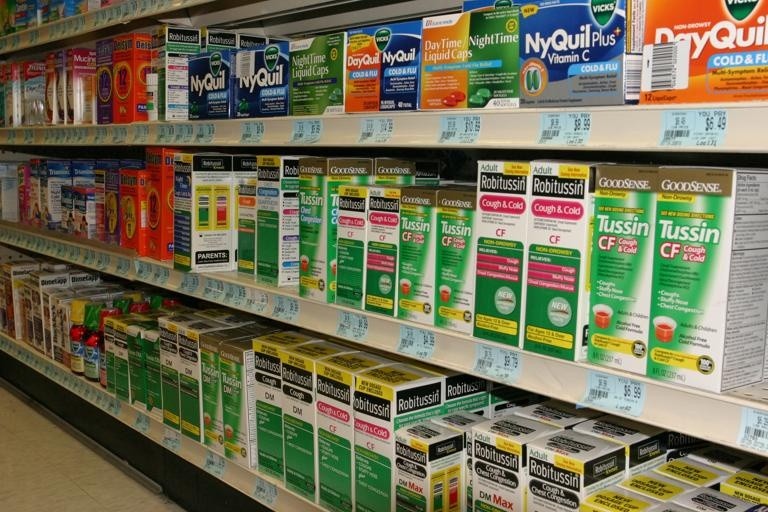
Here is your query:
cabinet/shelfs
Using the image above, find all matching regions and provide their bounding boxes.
[0,0,768,512]
